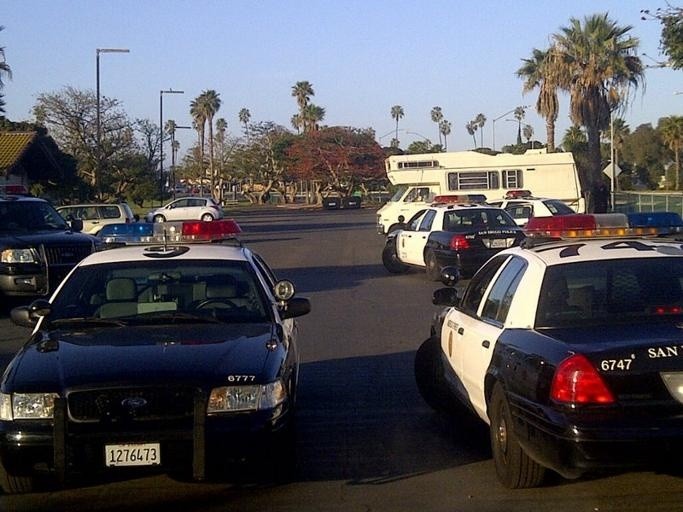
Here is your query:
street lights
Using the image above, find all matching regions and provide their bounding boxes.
[158,89,189,210]
[674,92,683,96]
[505,118,536,154]
[168,119,193,201]
[376,128,404,150]
[490,107,524,157]
[93,45,131,202]
[404,130,433,154]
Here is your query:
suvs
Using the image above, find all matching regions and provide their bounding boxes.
[0,191,104,311]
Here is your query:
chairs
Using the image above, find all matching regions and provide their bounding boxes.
[92,277,138,319]
[190,275,250,310]
[471,218,487,230]
[540,274,583,322]
[602,266,642,316]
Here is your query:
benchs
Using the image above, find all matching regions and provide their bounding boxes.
[138,276,193,305]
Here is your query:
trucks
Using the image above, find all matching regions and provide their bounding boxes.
[373,146,586,235]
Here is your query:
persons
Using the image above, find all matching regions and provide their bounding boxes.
[407,189,423,202]
[586,176,610,216]
[354,186,362,209]
[262,183,269,203]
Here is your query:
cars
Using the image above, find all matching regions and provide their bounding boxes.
[382,194,528,282]
[486,191,575,227]
[142,196,225,223]
[0,216,316,495]
[413,211,683,490]
[163,178,211,196]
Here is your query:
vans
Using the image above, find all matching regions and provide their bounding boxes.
[43,203,136,243]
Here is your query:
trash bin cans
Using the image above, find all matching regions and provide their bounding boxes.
[592,183,610,213]
